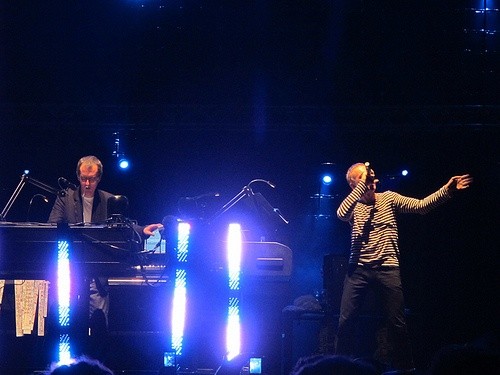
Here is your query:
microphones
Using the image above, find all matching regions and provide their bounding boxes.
[60,177,77,191]
[365,162,373,185]
[248,179,275,188]
[28,194,49,222]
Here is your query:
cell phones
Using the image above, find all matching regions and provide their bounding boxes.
[249,357,262,375]
[163,351,176,374]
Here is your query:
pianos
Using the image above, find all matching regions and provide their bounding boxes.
[0,216,158,287]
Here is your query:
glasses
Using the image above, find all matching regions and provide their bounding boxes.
[78,175,99,183]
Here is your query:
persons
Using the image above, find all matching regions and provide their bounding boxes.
[48,155,165,347]
[336,162,473,375]
